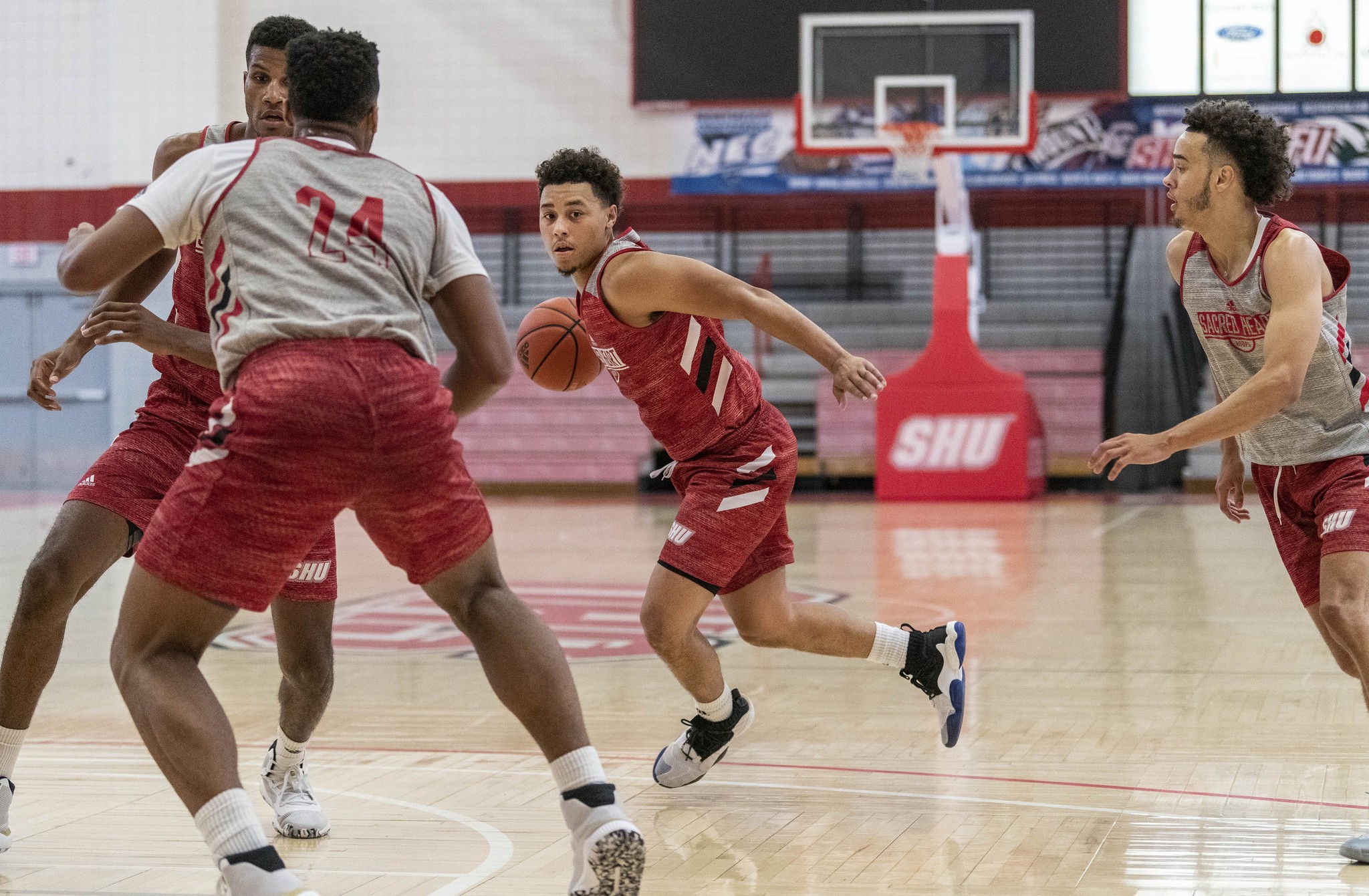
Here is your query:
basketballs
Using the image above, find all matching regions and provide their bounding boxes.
[515,297,605,392]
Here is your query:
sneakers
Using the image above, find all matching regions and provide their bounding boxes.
[0,776,15,853]
[652,692,754,789]
[257,740,331,838]
[217,846,322,896]
[909,621,966,748]
[559,796,646,896]
[654,807,757,893]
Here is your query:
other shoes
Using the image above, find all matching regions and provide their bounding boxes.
[1338,832,1369,862]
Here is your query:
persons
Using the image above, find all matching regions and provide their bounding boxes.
[0,14,336,893]
[61,28,647,895]
[538,148,969,787]
[1089,100,1369,868]
